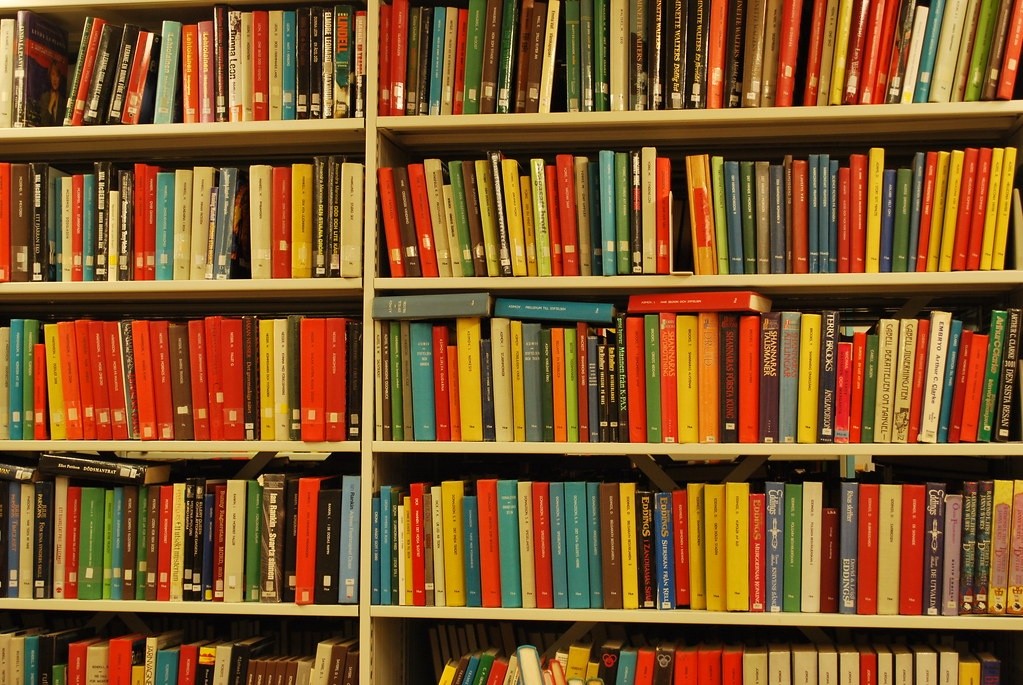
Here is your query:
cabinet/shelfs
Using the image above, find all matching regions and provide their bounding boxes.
[0,0,1023,685]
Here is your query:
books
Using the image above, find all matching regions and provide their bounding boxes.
[373,292,1023,443]
[378,146,1018,277]
[371,464,1023,614]
[380,0,1023,117]
[0,155,365,283]
[427,622,1000,685]
[0,4,367,127]
[0,317,365,440]
[0,615,359,685]
[0,454,360,604]
